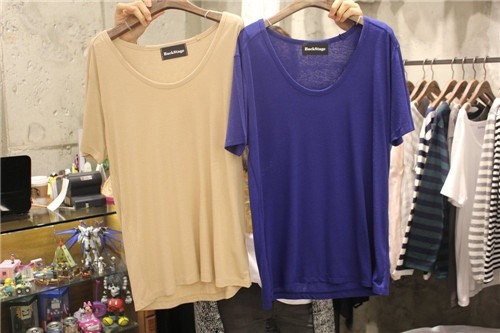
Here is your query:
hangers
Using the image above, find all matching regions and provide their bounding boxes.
[241,0,397,68]
[400,56,500,122]
[87,1,246,50]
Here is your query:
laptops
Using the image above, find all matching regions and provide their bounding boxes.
[0,155,32,215]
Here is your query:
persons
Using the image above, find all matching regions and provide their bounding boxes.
[115,0,365,332]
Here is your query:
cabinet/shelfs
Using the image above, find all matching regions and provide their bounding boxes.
[0,204,145,333]
